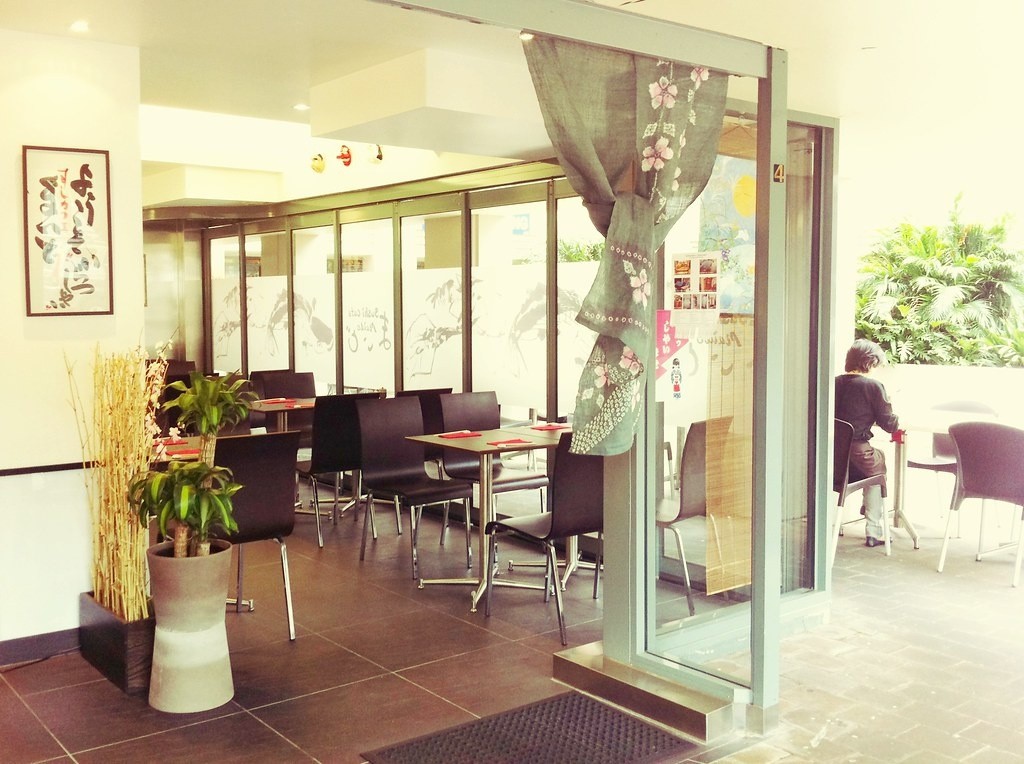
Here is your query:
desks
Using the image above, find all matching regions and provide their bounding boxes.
[890,409,995,549]
[248,397,316,433]
[147,435,216,464]
[403,423,604,613]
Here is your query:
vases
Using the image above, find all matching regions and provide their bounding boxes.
[79,591,157,697]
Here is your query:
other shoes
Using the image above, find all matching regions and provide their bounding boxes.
[860,506,865,515]
[867,537,893,547]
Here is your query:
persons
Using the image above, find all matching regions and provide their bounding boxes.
[836,338,900,547]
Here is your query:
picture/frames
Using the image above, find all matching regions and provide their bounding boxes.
[22,145,114,317]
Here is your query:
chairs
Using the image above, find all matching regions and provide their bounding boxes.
[937,422,1024,588]
[146,359,728,643]
[829,418,891,568]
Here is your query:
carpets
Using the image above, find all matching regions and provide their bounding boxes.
[360,690,698,764]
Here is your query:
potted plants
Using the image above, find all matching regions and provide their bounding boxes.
[127,368,262,714]
